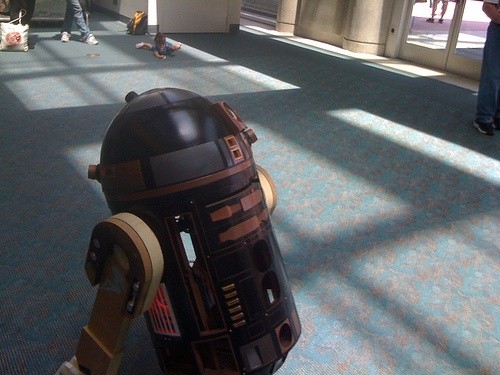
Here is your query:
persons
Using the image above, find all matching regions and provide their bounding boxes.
[9,0,35,49]
[136,32,180,59]
[426,0,448,24]
[0,0,8,13]
[60,0,99,45]
[473,0,500,136]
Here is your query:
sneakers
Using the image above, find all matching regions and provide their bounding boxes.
[472,117,500,136]
[60,32,71,43]
[84,34,99,46]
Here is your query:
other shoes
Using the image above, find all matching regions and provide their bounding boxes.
[438,19,443,23]
[135,42,144,49]
[27,39,35,49]
[427,18,435,23]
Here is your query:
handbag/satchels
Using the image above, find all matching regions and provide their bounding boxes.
[1,10,29,52]
